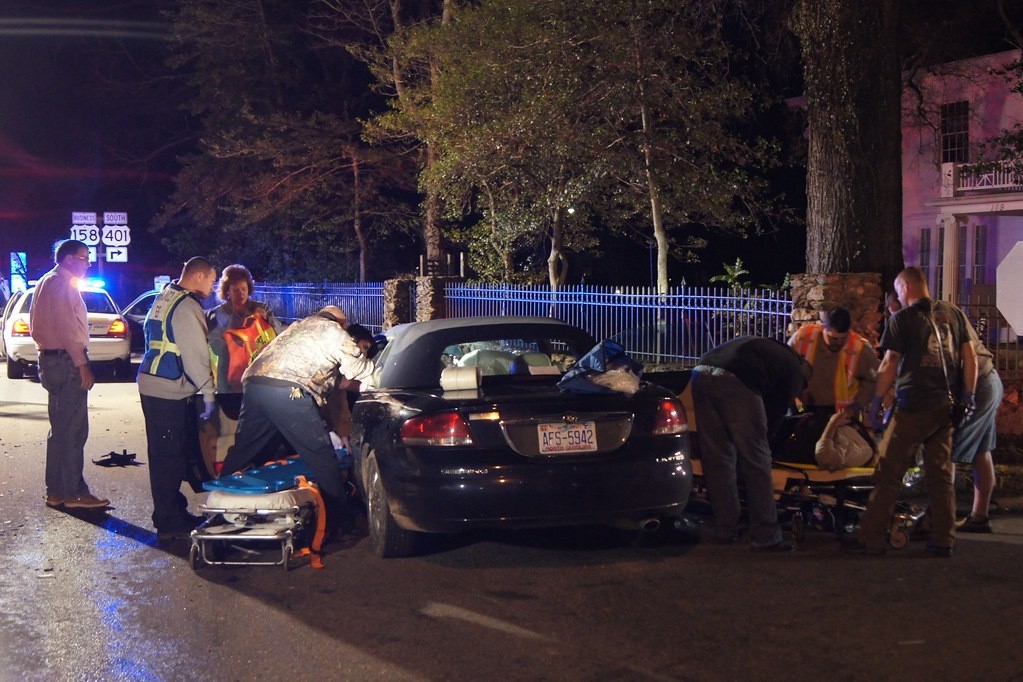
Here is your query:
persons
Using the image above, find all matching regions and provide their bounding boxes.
[839,266,979,558]
[31,239,111,510]
[136,256,219,540]
[208,264,282,394]
[219,304,376,540]
[881,286,1004,532]
[784,307,878,421]
[691,334,814,553]
[319,322,376,455]
[687,407,886,471]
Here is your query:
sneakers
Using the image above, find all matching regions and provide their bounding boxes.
[48,492,64,505]
[64,495,110,509]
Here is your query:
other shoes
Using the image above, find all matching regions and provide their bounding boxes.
[954,513,992,533]
[753,538,796,553]
[716,527,743,544]
[925,538,953,556]
[840,536,882,556]
[157,524,195,541]
[330,524,356,541]
[195,540,226,569]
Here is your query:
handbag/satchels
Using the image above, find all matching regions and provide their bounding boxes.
[944,391,962,417]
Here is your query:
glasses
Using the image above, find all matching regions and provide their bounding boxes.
[66,253,89,262]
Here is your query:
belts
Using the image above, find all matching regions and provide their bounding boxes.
[39,348,68,354]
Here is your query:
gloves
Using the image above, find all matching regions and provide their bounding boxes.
[869,394,884,429]
[959,392,977,420]
[200,401,216,421]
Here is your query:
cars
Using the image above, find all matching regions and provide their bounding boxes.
[0,277,162,381]
[349,314,694,560]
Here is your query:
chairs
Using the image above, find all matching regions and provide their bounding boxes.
[511,353,552,374]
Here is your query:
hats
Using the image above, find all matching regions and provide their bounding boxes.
[318,305,345,325]
